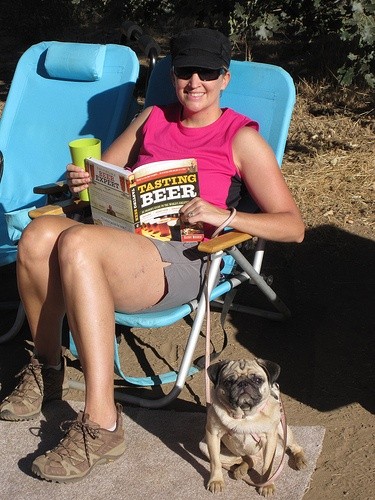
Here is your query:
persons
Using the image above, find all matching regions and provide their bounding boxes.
[0,27,305,484]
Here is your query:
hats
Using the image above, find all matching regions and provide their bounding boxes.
[169,27,233,70]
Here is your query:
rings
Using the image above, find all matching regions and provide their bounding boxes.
[188,211,194,217]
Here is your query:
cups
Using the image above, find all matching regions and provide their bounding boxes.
[68,138,101,202]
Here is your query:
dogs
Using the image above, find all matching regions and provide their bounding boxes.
[203,357,310,498]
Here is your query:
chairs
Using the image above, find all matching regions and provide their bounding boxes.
[0,40,139,349]
[28,58,297,408]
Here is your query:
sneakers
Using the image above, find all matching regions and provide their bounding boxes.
[30,402,129,484]
[1,354,66,420]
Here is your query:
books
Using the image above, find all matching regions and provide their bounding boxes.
[84,156,200,241]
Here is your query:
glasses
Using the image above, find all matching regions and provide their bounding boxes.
[175,67,226,81]
